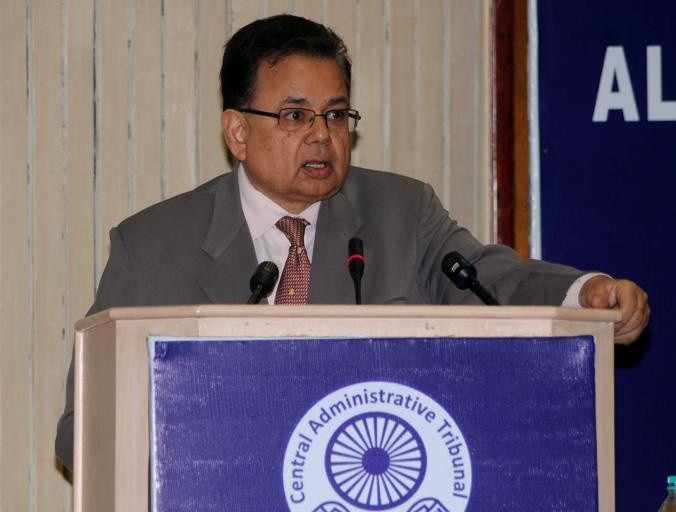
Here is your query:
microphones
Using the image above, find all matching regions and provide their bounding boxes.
[441,252,500,305]
[349,238,363,304]
[247,261,279,304]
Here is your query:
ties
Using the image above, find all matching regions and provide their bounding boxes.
[274,215,312,305]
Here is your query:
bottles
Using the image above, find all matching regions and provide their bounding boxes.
[656,474,675,512]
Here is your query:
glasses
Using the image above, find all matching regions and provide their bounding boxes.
[238,108,361,132]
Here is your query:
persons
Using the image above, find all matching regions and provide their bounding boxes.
[55,14,651,488]
